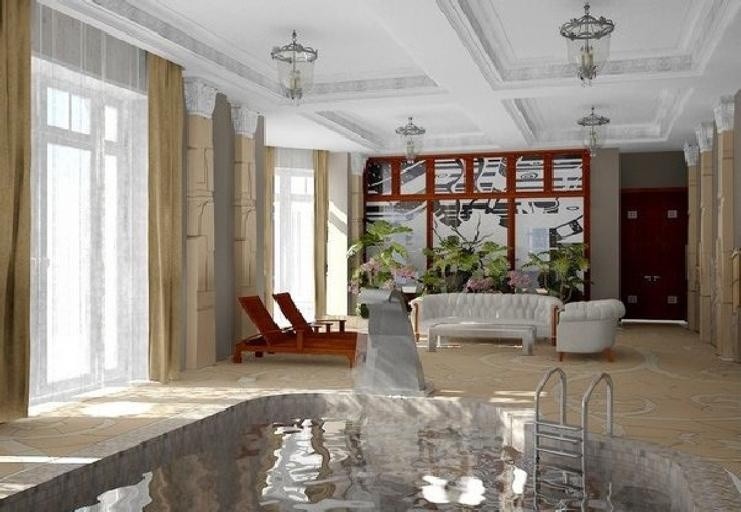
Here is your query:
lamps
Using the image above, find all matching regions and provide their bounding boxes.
[559,0,614,87]
[394,118,426,164]
[578,109,608,159]
[271,30,318,103]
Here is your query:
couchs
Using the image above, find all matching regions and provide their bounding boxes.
[407,293,561,347]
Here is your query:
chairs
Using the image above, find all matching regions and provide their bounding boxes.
[233,297,355,367]
[556,297,626,362]
[274,293,358,342]
